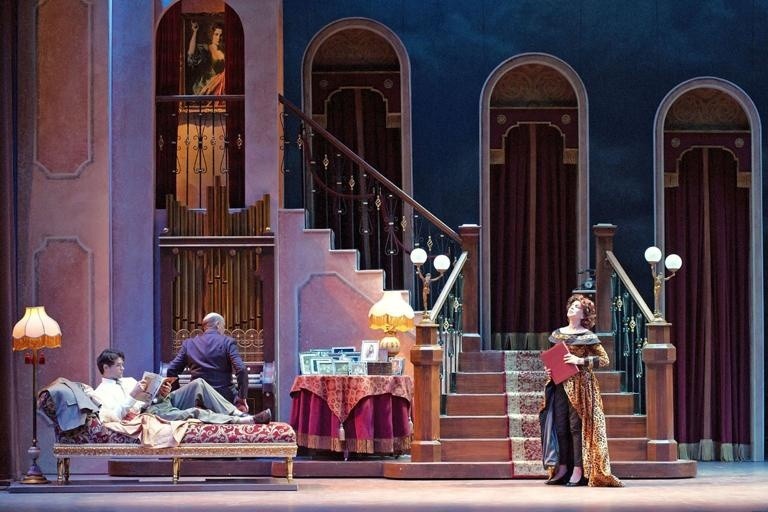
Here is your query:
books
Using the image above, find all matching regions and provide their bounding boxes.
[134,370,178,404]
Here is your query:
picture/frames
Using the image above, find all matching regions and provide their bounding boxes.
[298,340,405,375]
[179,11,226,114]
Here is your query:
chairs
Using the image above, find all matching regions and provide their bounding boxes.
[37,388,298,486]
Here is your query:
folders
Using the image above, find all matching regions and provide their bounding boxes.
[539,341,579,385]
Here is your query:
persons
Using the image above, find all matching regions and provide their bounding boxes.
[365,344,374,359]
[92,348,272,426]
[417,266,443,315]
[166,312,250,413]
[538,293,626,488]
[186,18,226,106]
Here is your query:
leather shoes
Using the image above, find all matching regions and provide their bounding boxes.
[544,470,572,485]
[567,471,583,486]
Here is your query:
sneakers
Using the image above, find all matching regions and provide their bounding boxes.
[253,408,272,424]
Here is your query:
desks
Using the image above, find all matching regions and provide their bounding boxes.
[290,375,415,461]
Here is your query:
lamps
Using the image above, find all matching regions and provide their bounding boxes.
[410,248,450,324]
[12,306,62,485]
[644,246,683,323]
[368,292,415,375]
